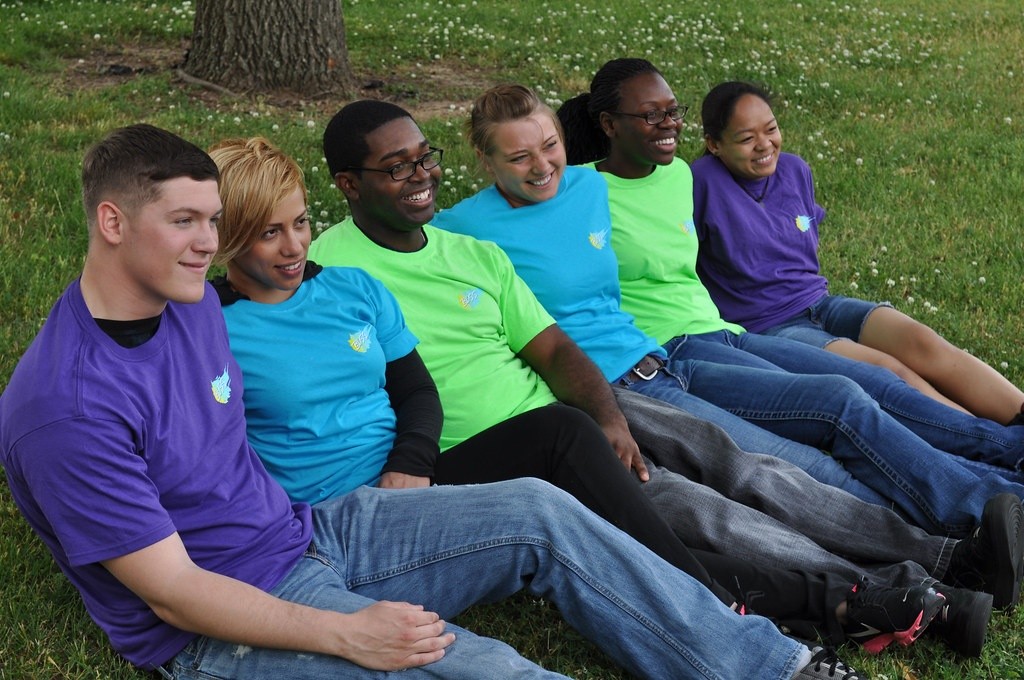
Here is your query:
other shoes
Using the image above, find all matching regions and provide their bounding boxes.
[789,646,863,680]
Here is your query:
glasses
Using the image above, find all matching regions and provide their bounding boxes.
[348,147,445,181]
[608,105,689,125]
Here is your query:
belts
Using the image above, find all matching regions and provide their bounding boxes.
[619,355,663,388]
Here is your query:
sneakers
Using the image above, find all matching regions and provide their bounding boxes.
[843,576,943,656]
[928,581,994,660]
[739,604,827,661]
[961,491,1024,617]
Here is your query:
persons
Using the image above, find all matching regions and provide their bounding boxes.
[1,125,896,680]
[207,137,948,655]
[306,100,1021,657]
[691,81,1022,426]
[557,58,1024,484]
[425,84,1023,537]
